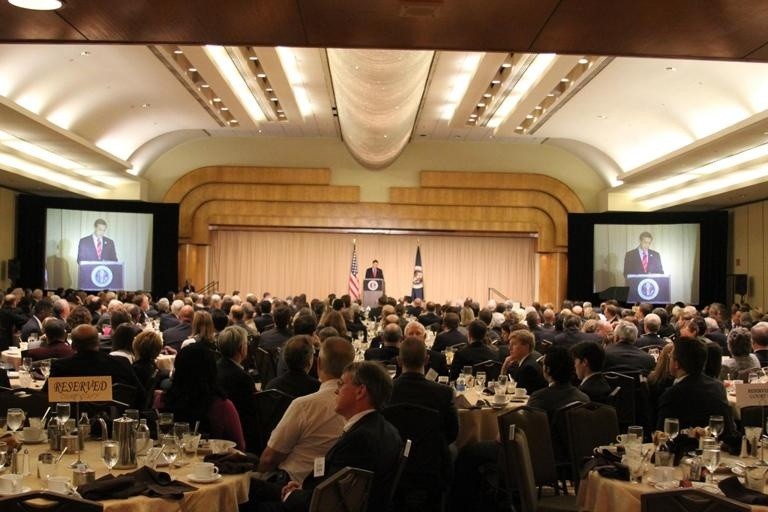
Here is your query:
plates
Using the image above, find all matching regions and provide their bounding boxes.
[188,473,223,483]
[491,401,510,406]
[198,438,248,464]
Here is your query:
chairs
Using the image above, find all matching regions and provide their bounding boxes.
[2,309,411,512]
[421,329,768,512]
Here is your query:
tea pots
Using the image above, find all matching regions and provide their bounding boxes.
[98,414,151,470]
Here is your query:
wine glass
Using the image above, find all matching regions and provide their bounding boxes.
[8,332,44,355]
[443,348,457,362]
[351,327,382,363]
[437,366,508,395]
[627,416,766,504]
[17,356,51,391]
[724,368,768,397]
[648,349,661,362]
[0,403,122,496]
[151,409,205,484]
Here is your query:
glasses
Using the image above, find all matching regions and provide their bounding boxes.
[337,381,359,388]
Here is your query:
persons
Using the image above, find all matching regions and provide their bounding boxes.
[365,259,384,279]
[411,243,424,299]
[77,217,119,266]
[348,240,361,302]
[623,233,663,277]
[182,278,195,292]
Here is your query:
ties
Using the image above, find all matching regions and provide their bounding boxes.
[641,251,648,273]
[96,238,102,260]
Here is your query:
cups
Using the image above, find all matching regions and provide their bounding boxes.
[195,461,219,478]
[494,393,509,403]
[124,409,140,418]
[508,380,516,394]
[515,388,528,397]
[617,433,637,447]
[594,444,618,455]
[144,320,161,332]
